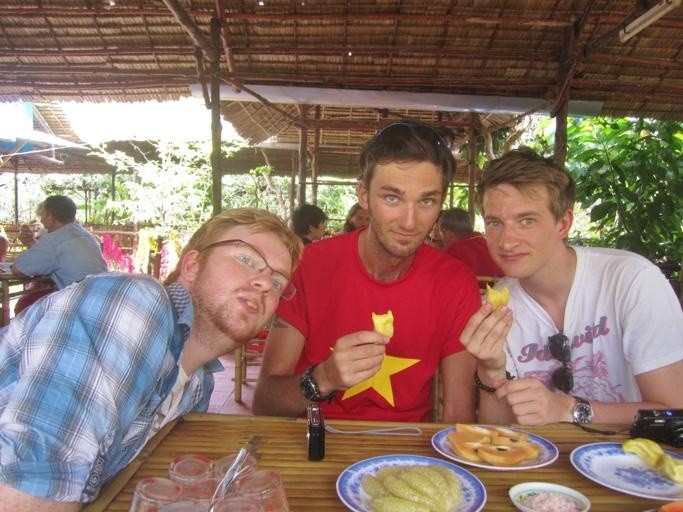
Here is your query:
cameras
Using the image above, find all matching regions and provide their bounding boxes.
[630,408,683,448]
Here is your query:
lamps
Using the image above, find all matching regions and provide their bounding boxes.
[619,0,682,43]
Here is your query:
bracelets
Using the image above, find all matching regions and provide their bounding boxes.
[472,370,514,394]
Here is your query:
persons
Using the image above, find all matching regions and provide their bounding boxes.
[341,203,370,232]
[424,211,447,250]
[11,195,108,317]
[437,208,503,290]
[288,203,329,245]
[250,119,481,425]
[13,202,59,316]
[457,144,683,428]
[0,206,304,512]
[0,235,8,274]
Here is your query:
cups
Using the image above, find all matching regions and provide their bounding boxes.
[123,448,291,512]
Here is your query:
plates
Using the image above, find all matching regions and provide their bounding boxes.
[568,442,683,501]
[430,423,560,470]
[334,453,487,512]
[508,481,591,512]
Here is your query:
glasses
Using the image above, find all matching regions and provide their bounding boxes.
[375,122,445,146]
[549,335,573,393]
[199,240,297,301]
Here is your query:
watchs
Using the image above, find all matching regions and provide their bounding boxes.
[572,395,593,426]
[299,364,338,404]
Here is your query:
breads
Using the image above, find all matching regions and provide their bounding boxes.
[450,424,541,467]
[485,283,511,310]
[623,437,683,483]
[371,310,395,338]
